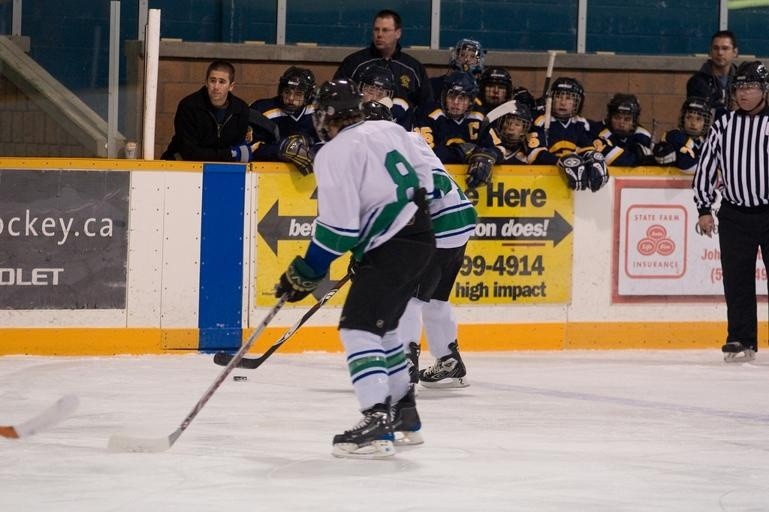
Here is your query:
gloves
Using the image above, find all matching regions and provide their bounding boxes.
[270,253,328,303]
[581,150,609,193]
[463,148,498,192]
[278,133,316,177]
[556,154,588,192]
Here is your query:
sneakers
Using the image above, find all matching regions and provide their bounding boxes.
[721,339,751,353]
[332,403,395,445]
[416,353,467,383]
[390,398,422,433]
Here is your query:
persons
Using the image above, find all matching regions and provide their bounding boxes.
[160,60,249,162]
[274,78,436,446]
[360,100,477,385]
[691,60,768,353]
[330,9,430,108]
[248,65,322,176]
[595,93,651,166]
[652,96,711,170]
[530,76,638,192]
[686,30,738,125]
[331,37,536,188]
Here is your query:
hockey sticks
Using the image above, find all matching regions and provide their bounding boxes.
[215,270,353,367]
[107,292,287,454]
[0,396,77,438]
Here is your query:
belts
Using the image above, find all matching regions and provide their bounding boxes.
[720,197,769,214]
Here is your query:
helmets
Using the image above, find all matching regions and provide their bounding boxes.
[604,92,642,140]
[550,76,585,119]
[496,101,535,150]
[441,70,479,122]
[315,77,364,126]
[677,97,714,141]
[356,59,396,100]
[727,60,767,85]
[276,65,317,117]
[479,67,512,107]
[448,37,486,75]
